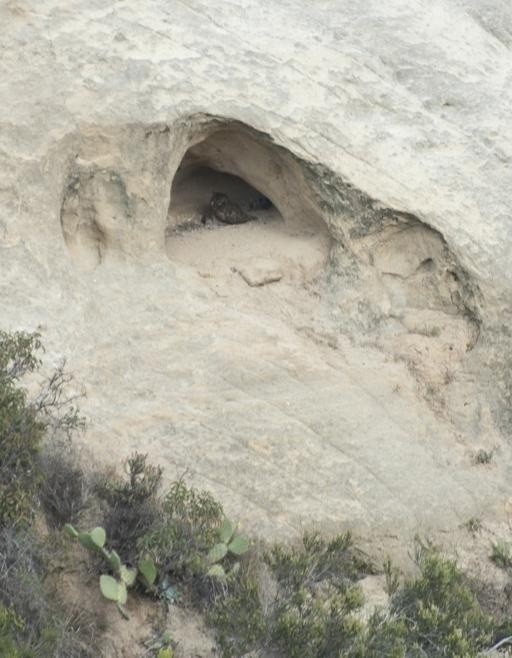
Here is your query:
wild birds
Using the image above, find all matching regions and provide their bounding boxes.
[200,192,257,225]
